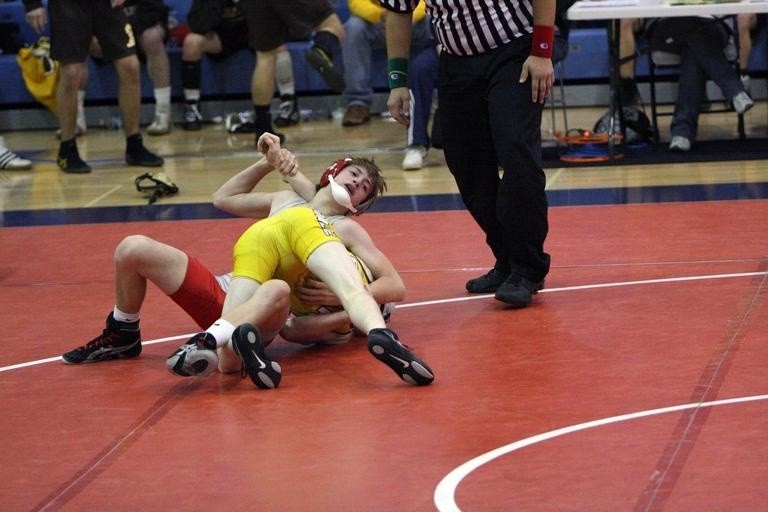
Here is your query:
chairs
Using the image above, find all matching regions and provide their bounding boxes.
[647,47,750,142]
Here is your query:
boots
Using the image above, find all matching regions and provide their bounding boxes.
[60,312,141,365]
[165,332,218,377]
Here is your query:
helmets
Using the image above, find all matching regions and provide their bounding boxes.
[320,157,375,216]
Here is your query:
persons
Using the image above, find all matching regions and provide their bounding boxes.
[60,147,409,376]
[215,132,436,390]
[590,0,758,156]
[736,11,766,89]
[1,0,449,170]
[383,1,555,307]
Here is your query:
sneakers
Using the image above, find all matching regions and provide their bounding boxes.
[125,145,163,167]
[146,104,172,134]
[669,136,689,151]
[732,92,753,114]
[57,109,86,138]
[0,143,33,170]
[226,99,255,132]
[341,102,369,124]
[56,150,90,174]
[182,101,200,130]
[366,328,434,386]
[230,324,280,390]
[402,143,427,169]
[464,269,537,306]
[307,46,346,93]
[275,101,300,125]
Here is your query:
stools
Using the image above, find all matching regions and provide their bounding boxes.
[545,62,570,137]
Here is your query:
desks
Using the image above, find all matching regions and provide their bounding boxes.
[566,1,768,164]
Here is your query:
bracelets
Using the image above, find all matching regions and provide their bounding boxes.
[529,22,555,60]
[385,58,410,92]
